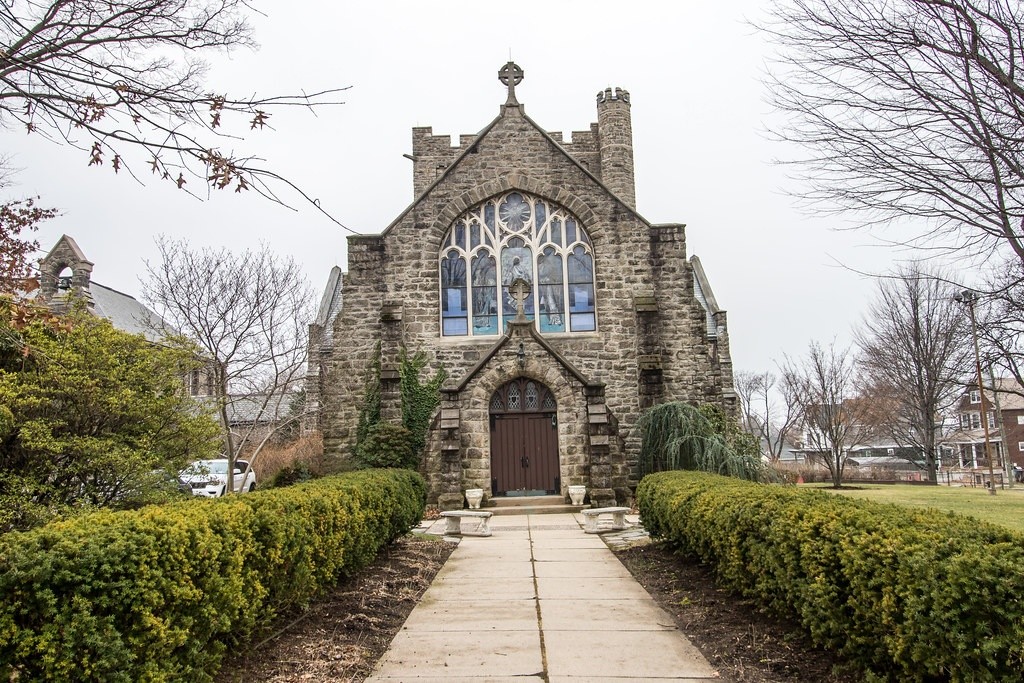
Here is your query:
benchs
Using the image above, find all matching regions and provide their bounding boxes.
[582,506,630,534]
[439,512,494,537]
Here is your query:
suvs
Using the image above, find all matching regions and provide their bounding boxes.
[168,458,257,499]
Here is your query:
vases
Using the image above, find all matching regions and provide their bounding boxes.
[568,485,586,506]
[465,490,484,509]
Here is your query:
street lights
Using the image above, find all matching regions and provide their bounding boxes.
[952,289,997,496]
